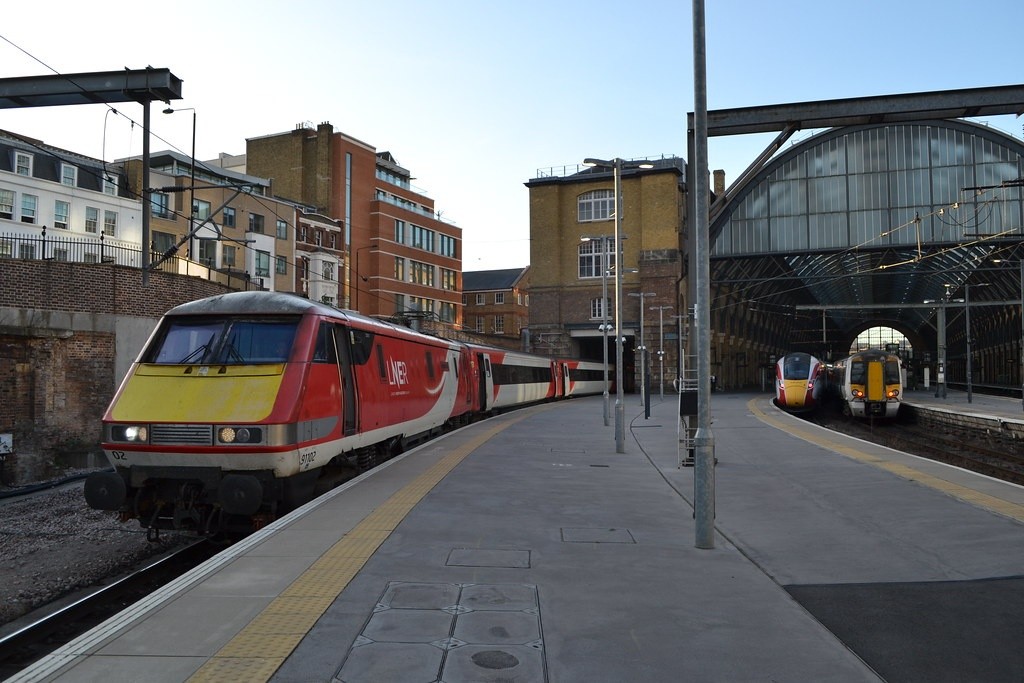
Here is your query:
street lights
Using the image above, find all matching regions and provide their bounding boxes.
[580,233,610,427]
[162,106,196,259]
[944,283,990,404]
[582,156,626,454]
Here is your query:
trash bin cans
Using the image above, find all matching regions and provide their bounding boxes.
[710,376,716,392]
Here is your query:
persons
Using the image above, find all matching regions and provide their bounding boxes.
[273,340,289,357]
[711,372,718,394]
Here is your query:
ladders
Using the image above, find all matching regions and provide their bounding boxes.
[676,348,698,469]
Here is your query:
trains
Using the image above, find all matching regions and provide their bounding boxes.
[81,290,619,545]
[774,352,833,417]
[827,347,906,423]
